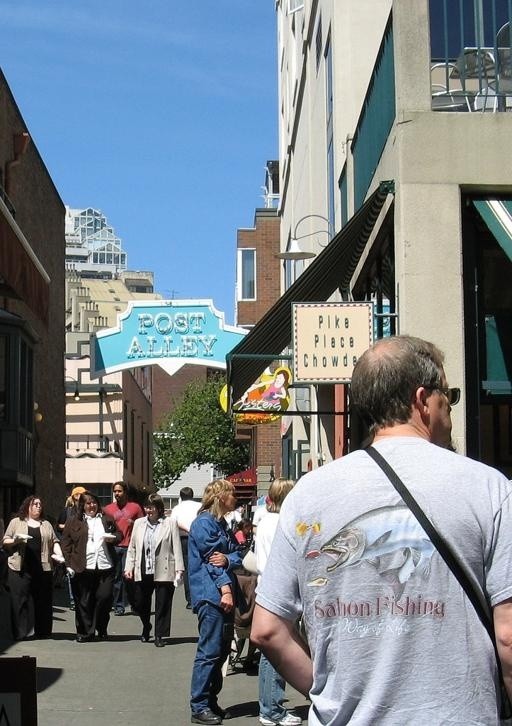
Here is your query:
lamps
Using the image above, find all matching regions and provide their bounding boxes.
[274,213,329,263]
[66,375,84,404]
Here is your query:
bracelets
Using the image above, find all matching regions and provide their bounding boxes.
[221,592,232,597]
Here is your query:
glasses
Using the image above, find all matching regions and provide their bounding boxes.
[414,384,461,406]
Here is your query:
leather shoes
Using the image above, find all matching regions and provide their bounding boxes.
[77,635,96,641]
[213,705,231,719]
[191,709,222,724]
[155,639,164,647]
[141,623,152,641]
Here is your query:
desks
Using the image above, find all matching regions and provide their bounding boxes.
[449,47,512,112]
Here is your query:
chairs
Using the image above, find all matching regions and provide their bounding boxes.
[430,62,475,112]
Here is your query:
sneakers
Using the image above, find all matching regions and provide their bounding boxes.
[259,711,302,726]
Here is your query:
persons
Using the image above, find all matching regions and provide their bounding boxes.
[254,477,301,726]
[102,481,144,616]
[61,491,124,642]
[66,487,89,611]
[171,487,203,609]
[2,496,65,641]
[124,494,185,647]
[250,336,512,726]
[188,479,246,725]
